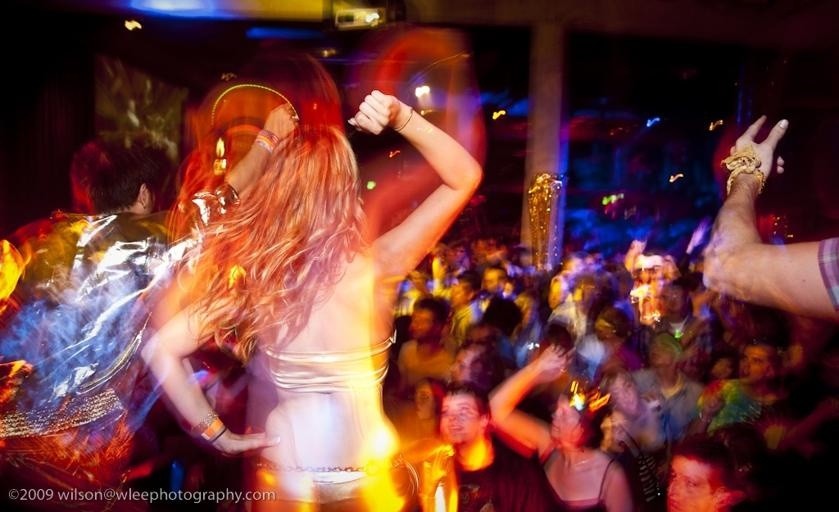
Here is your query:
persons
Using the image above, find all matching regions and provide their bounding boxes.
[701,112,839,327]
[138,88,488,511]
[0,100,301,510]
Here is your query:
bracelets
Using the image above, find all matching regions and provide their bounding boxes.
[252,126,279,159]
[718,144,769,194]
[191,412,232,449]
[391,108,417,133]
